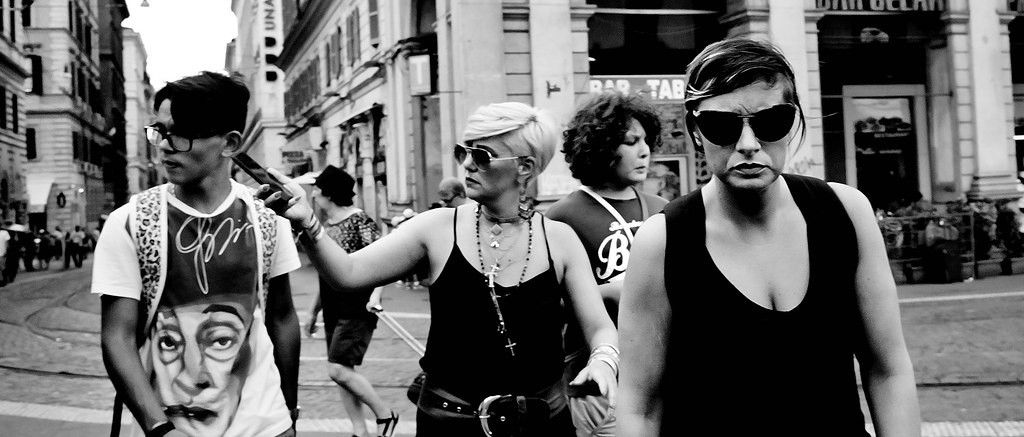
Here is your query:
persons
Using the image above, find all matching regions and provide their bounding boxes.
[540,92,670,437]
[614,37,921,437]
[91,70,302,437]
[252,101,619,437]
[302,164,401,437]
[875,170,1024,285]
[391,176,477,289]
[0,219,101,287]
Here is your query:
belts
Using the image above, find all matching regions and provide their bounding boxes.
[423,390,570,424]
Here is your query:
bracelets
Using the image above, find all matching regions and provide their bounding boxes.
[291,208,326,248]
[586,343,620,375]
[145,420,175,437]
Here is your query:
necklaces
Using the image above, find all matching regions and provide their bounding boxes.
[476,203,533,356]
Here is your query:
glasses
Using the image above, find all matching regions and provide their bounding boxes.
[145,123,192,154]
[453,144,527,172]
[691,103,797,147]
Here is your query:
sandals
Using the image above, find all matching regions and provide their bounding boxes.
[377,411,399,437]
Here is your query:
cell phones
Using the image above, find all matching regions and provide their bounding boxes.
[231,152,293,203]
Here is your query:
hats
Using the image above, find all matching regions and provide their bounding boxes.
[313,165,357,198]
